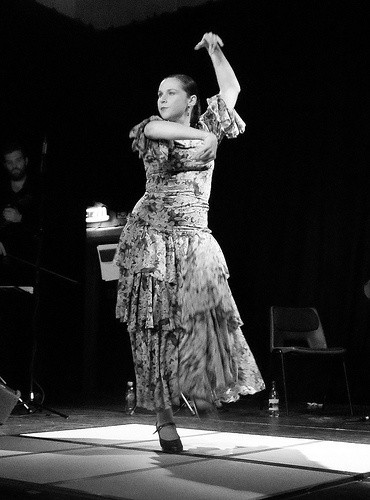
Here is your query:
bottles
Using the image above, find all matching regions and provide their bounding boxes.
[268,382,280,418]
[126,381,137,417]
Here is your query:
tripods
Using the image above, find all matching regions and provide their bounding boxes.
[19,259,78,419]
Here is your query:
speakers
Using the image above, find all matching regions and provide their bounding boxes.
[0,381,19,426]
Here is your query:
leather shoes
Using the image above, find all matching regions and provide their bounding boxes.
[153,423,183,452]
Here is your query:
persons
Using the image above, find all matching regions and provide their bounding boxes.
[0,142,40,385]
[111,32,266,453]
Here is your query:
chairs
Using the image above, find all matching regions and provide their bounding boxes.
[270,305,354,417]
